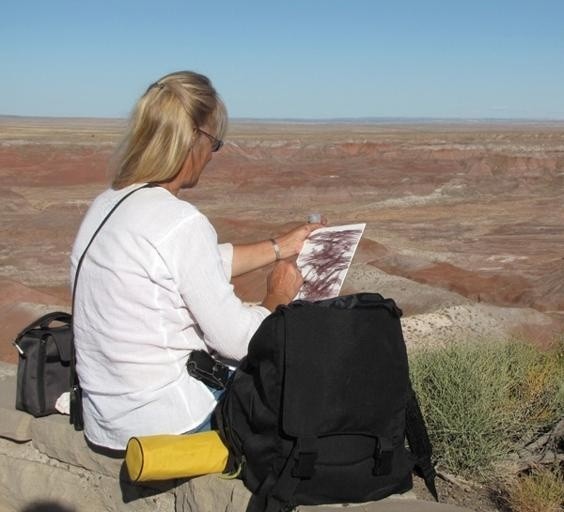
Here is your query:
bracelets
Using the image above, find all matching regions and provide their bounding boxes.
[267,238,280,261]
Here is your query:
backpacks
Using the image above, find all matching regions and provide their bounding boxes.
[187,289,438,511]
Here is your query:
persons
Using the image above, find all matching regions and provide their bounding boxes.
[68,71,331,459]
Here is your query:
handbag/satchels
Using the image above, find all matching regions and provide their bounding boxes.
[12,310,73,418]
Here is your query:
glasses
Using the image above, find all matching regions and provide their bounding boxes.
[196,128,225,153]
